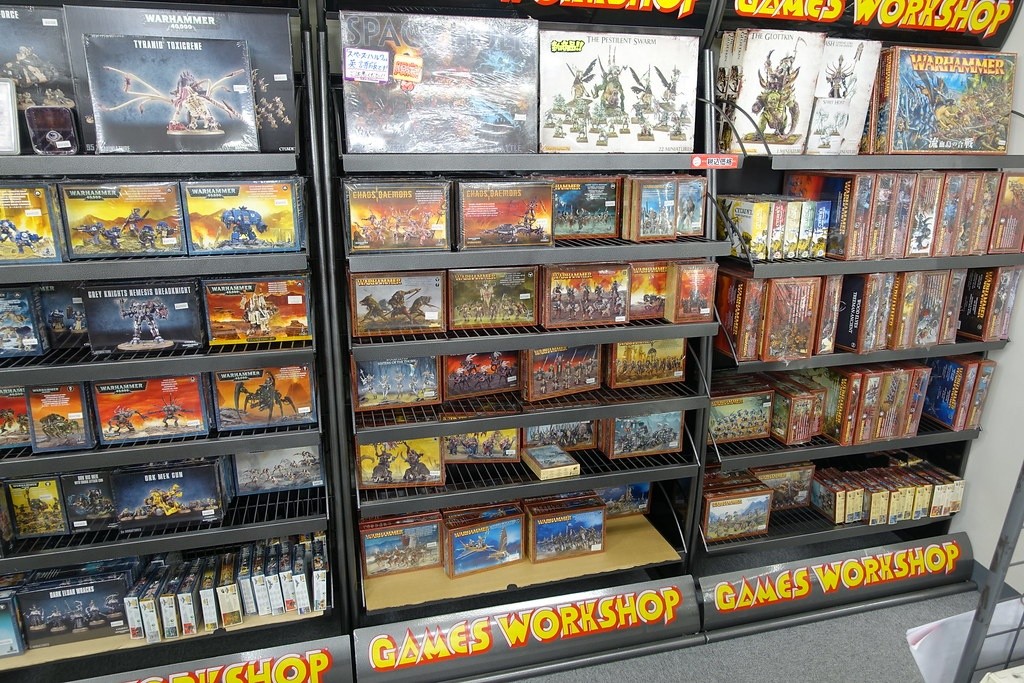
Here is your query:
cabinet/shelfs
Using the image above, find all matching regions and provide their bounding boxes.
[316,0,717,683]
[0,0,352,682]
[692,1,1023,644]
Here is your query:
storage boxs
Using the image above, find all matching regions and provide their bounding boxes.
[0,5,1024,656]
